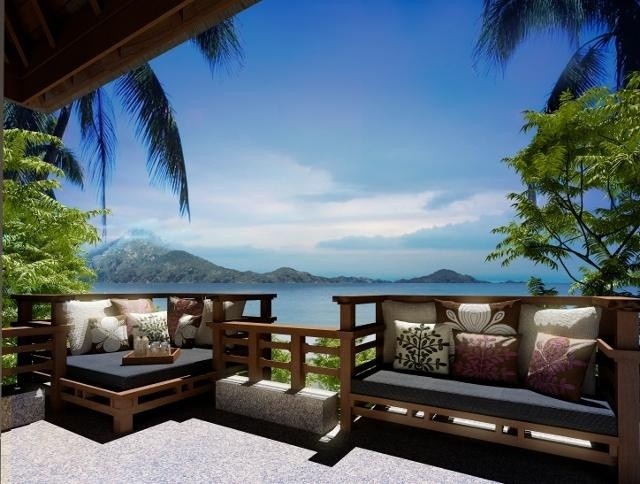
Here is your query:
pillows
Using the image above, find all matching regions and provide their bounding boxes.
[62,299,245,353]
[392,298,601,404]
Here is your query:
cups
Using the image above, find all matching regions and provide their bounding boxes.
[133,329,150,358]
[150,341,171,357]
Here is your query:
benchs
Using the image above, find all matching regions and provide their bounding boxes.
[332,294,640,484]
[9,293,276,432]
